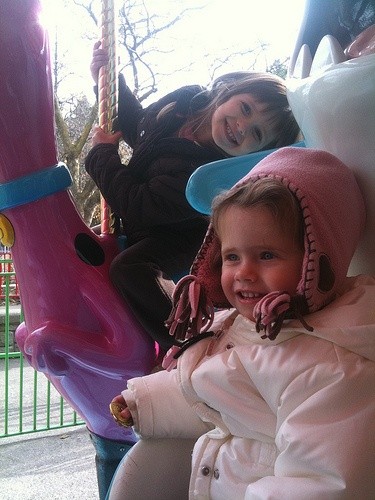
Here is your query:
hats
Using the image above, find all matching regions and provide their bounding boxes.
[167,147,366,341]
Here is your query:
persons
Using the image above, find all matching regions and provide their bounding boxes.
[84,38,375,500]
